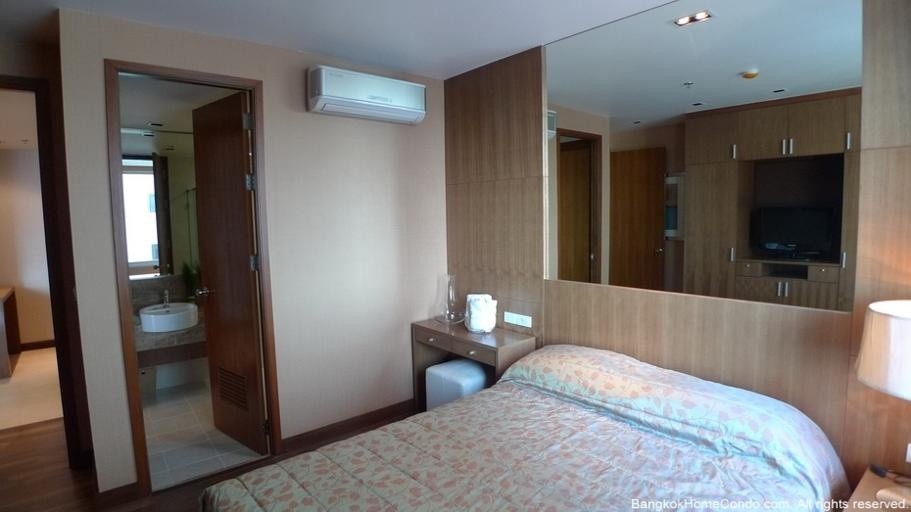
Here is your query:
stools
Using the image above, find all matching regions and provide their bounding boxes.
[424,359,488,411]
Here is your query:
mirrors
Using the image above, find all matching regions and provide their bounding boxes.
[120,154,174,282]
[546,0,864,316]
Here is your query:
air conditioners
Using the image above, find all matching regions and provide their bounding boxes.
[307,65,427,127]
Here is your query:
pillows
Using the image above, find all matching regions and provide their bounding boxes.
[504,345,830,485]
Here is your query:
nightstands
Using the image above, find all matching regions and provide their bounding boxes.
[413,316,536,414]
[842,467,911,512]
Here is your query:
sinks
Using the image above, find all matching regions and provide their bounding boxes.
[139,302,199,333]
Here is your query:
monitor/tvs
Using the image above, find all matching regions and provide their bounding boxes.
[748,153,844,260]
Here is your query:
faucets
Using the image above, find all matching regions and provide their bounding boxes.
[161,287,171,308]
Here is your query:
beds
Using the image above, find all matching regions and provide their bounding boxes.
[206,375,838,512]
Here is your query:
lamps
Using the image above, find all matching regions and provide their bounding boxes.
[854,299,911,400]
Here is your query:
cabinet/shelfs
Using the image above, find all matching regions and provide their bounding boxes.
[681,87,861,313]
